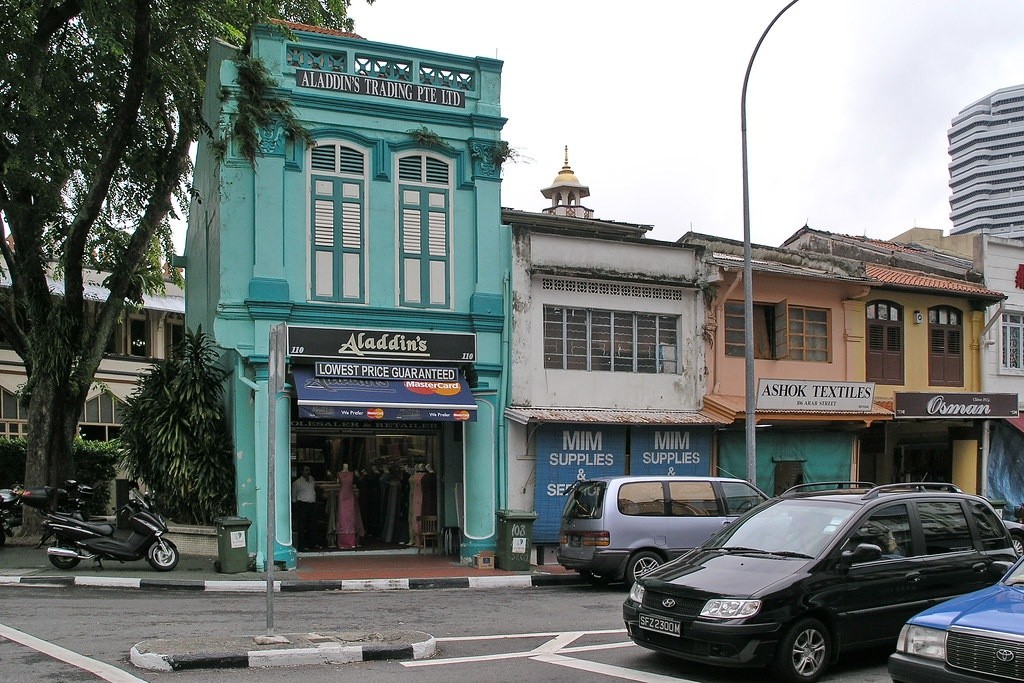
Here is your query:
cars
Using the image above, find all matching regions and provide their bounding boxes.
[888,556,1024,683]
[1001,520,1024,558]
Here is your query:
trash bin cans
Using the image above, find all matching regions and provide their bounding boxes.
[494,506,536,574]
[212,516,257,574]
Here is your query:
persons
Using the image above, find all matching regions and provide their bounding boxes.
[337,463,356,550]
[291,466,322,552]
[360,464,438,547]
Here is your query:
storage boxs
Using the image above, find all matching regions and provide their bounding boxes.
[472,550,496,570]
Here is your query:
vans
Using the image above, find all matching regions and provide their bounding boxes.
[556,475,772,590]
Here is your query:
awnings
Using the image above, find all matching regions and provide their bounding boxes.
[292,366,477,423]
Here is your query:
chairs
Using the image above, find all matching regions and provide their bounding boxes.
[416,514,441,556]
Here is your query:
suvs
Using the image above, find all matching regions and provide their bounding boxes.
[622,482,1019,683]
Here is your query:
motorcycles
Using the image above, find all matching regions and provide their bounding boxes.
[73,484,96,517]
[0,489,22,546]
[11,483,23,525]
[23,487,179,572]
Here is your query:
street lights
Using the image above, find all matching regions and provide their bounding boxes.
[741,1,799,482]
